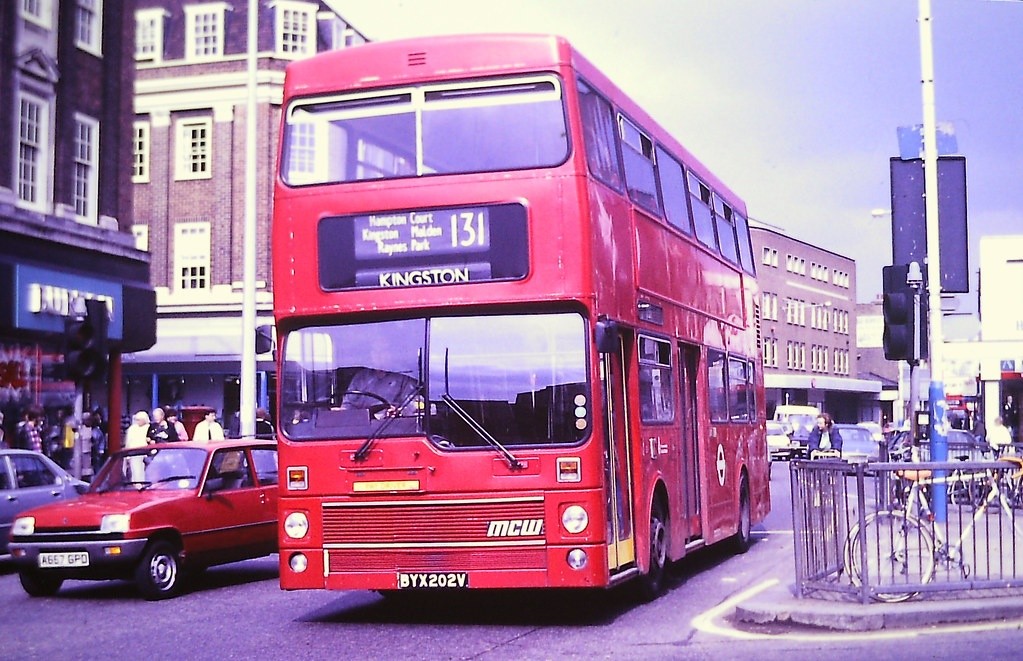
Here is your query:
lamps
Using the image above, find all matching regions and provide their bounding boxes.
[781,300,832,311]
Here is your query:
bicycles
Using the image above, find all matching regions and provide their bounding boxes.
[843,455,1023,603]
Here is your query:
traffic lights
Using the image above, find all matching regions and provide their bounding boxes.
[882,285,920,365]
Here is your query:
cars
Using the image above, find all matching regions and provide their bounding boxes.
[6,439,278,600]
[0,449,93,554]
[835,421,989,463]
[764,420,814,462]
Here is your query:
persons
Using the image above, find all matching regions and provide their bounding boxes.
[807,412,844,460]
[985,396,1018,460]
[340,334,424,417]
[0,406,278,489]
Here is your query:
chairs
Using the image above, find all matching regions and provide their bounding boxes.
[222,478,251,490]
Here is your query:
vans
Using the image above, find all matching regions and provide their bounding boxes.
[773,405,820,423]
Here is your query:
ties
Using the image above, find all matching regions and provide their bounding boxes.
[208,429,211,440]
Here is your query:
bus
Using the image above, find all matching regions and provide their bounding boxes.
[271,32,771,607]
